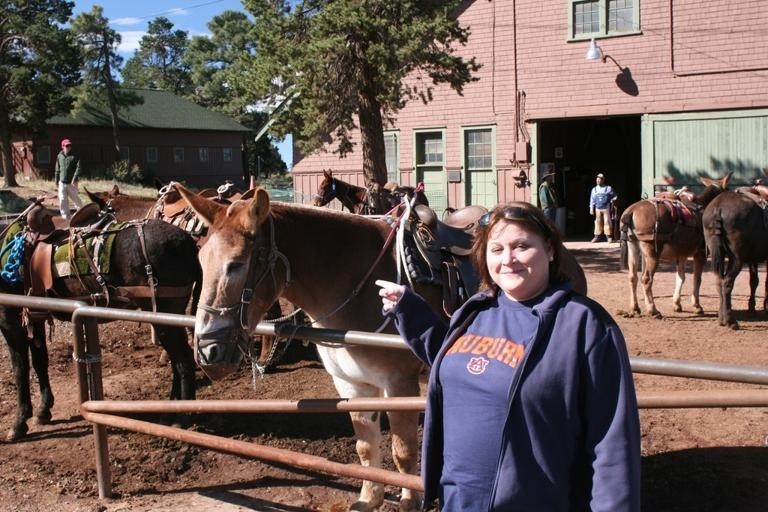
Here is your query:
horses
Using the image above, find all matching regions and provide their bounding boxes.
[1,221,204,443]
[83,183,284,376]
[171,182,588,512]
[701,168,768,331]
[314,169,434,220]
[620,171,735,320]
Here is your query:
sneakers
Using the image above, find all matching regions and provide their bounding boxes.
[607,237,614,242]
[591,235,601,242]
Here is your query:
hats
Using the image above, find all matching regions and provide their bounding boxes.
[596,174,605,178]
[540,168,557,180]
[61,138,73,147]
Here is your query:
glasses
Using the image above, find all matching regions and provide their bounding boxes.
[477,206,540,227]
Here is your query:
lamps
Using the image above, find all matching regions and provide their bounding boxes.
[586,38,606,62]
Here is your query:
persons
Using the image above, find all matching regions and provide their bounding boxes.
[538,168,560,229]
[587,172,618,244]
[54,139,85,221]
[373,199,643,509]
